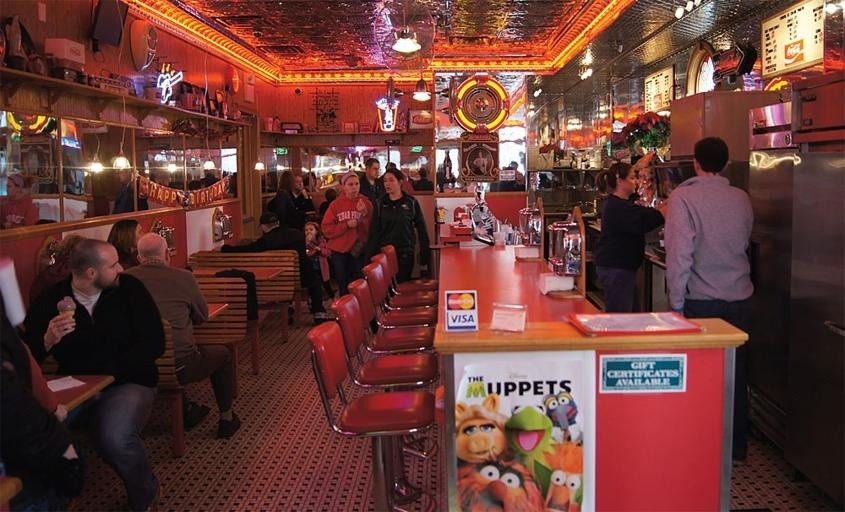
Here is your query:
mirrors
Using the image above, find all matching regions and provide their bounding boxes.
[289,146,311,196]
[62,137,91,202]
[0,131,57,201]
[0,108,62,233]
[388,145,434,196]
[433,70,529,198]
[134,124,185,215]
[59,113,135,227]
[184,131,241,215]
[260,143,290,199]
[309,145,388,196]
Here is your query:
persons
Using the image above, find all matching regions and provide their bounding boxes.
[304,221,338,309]
[23,236,166,511]
[303,172,320,191]
[268,170,297,229]
[595,161,665,313]
[369,169,429,335]
[664,136,753,466]
[386,162,413,194]
[293,176,318,221]
[411,168,434,191]
[1,174,39,230]
[107,219,143,269]
[213,214,339,321]
[0,288,86,512]
[509,161,525,184]
[321,173,373,297]
[360,158,383,203]
[437,171,453,193]
[121,233,240,437]
[112,169,150,214]
[319,188,338,213]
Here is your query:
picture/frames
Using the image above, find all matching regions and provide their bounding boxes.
[759,1,823,80]
[644,63,675,117]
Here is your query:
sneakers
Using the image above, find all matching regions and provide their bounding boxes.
[217,411,241,438]
[184,401,210,429]
[148,485,163,512]
[313,306,334,324]
[329,289,338,299]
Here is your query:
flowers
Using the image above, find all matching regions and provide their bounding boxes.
[612,110,673,150]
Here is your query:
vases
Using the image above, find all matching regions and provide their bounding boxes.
[639,146,670,163]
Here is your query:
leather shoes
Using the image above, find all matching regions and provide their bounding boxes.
[732,448,749,460]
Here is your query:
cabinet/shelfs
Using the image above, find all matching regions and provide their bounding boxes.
[0,66,254,131]
[526,169,608,212]
[260,129,415,143]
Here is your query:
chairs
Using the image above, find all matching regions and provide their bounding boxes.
[382,243,437,292]
[308,318,437,512]
[372,252,438,310]
[348,277,436,355]
[363,263,437,329]
[331,294,438,463]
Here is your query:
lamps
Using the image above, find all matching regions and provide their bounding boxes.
[83,133,105,173]
[411,54,432,103]
[376,96,401,132]
[391,0,422,54]
[167,162,178,173]
[203,136,216,170]
[111,128,132,171]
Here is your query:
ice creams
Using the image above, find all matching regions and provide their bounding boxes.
[57,296,76,330]
[307,232,313,241]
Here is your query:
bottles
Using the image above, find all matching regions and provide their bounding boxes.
[443,150,453,181]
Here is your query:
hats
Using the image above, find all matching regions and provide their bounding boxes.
[257,212,280,228]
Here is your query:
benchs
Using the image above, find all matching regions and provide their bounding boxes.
[248,310,269,375]
[197,250,307,330]
[154,319,193,458]
[189,276,249,400]
[187,252,297,343]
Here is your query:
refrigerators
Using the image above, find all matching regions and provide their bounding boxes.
[740,73,843,509]
